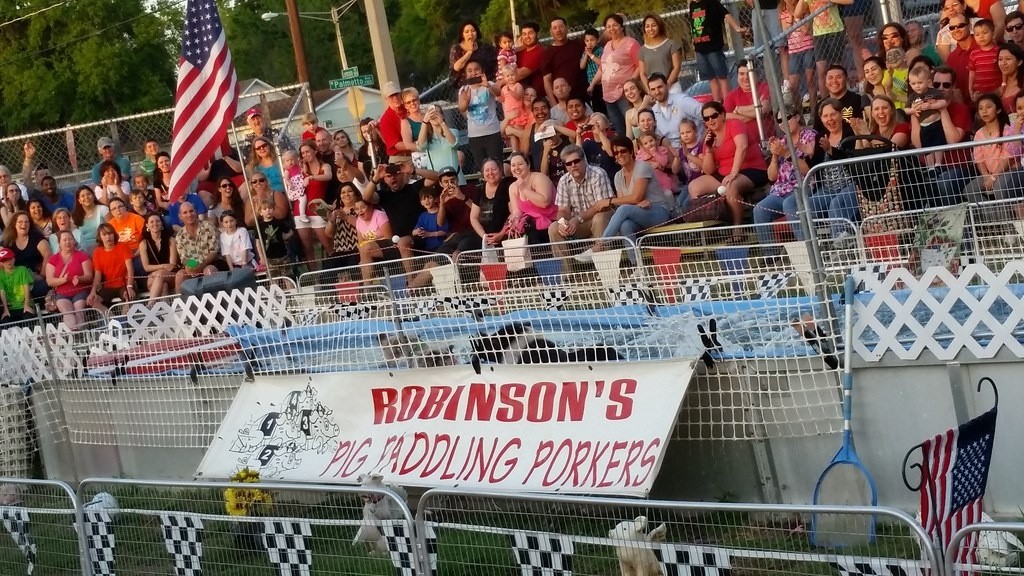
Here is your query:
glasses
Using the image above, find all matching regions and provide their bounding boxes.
[110,204,126,211]
[613,147,632,154]
[404,99,419,105]
[565,157,584,166]
[8,188,21,194]
[251,177,266,184]
[220,184,233,188]
[254,142,268,151]
[932,81,952,89]
[776,114,793,122]
[1005,22,1024,33]
[948,22,968,31]
[703,112,722,120]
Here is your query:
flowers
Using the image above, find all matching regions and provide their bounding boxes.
[223,466,279,517]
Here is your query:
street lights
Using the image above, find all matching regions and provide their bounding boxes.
[261,0,364,144]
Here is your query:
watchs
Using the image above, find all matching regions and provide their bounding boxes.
[577,216,584,223]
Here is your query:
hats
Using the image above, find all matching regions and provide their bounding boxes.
[97,137,115,154]
[246,108,262,121]
[383,82,401,97]
[0,248,15,261]
[438,165,457,182]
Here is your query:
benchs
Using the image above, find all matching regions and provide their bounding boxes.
[630,219,723,260]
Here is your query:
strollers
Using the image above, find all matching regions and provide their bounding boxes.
[836,135,923,207]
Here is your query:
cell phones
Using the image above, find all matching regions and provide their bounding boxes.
[335,152,343,160]
[386,164,401,174]
[705,132,717,149]
[448,182,455,190]
[467,76,482,85]
[429,105,435,112]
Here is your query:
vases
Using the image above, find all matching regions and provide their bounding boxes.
[233,528,260,545]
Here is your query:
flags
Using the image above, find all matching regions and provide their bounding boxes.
[169,0,239,204]
[921,408,999,576]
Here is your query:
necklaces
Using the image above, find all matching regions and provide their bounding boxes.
[986,128,991,136]
[89,215,92,218]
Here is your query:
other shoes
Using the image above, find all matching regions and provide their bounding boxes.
[574,249,594,263]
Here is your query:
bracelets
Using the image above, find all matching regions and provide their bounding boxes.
[468,50,473,51]
[991,176,996,181]
[786,156,792,163]
[1001,84,1006,86]
[463,196,469,202]
[423,121,427,124]
[591,55,594,59]
[371,180,375,184]
[127,285,133,288]
[609,197,614,206]
[162,265,163,269]
[312,176,314,180]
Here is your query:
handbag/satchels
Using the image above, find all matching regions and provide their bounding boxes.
[478,234,501,288]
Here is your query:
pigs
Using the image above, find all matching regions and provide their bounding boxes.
[609,514,664,576]
[355,472,406,556]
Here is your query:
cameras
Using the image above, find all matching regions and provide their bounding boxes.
[581,124,593,132]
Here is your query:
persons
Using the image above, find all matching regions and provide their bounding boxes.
[0,0,1024,332]
